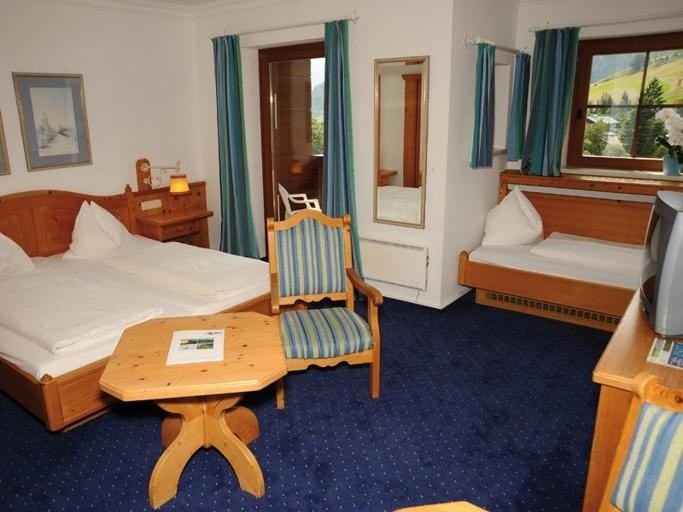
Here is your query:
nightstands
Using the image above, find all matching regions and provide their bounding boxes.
[379,169,398,187]
[137,208,213,248]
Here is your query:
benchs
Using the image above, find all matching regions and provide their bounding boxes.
[457,169,683,332]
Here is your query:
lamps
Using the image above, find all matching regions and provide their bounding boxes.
[137,159,190,194]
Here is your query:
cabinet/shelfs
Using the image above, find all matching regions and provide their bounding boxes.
[581,287,683,511]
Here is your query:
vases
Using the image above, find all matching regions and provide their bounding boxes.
[662,155,679,176]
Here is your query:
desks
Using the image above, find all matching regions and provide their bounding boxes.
[98,311,288,510]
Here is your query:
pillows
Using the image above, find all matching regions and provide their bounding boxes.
[482,185,544,248]
[0,201,134,281]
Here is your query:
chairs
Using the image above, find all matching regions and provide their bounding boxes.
[267,209,384,409]
[597,371,683,511]
[278,183,322,221]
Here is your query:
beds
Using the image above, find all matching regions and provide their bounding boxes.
[0,184,309,434]
[378,185,421,227]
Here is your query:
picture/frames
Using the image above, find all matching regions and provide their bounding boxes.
[0,111,11,176]
[12,72,92,172]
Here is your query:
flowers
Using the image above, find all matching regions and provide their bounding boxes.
[655,107,683,156]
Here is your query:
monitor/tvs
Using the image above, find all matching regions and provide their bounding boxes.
[637,189,683,340]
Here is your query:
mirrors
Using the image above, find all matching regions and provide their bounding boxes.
[374,55,429,229]
